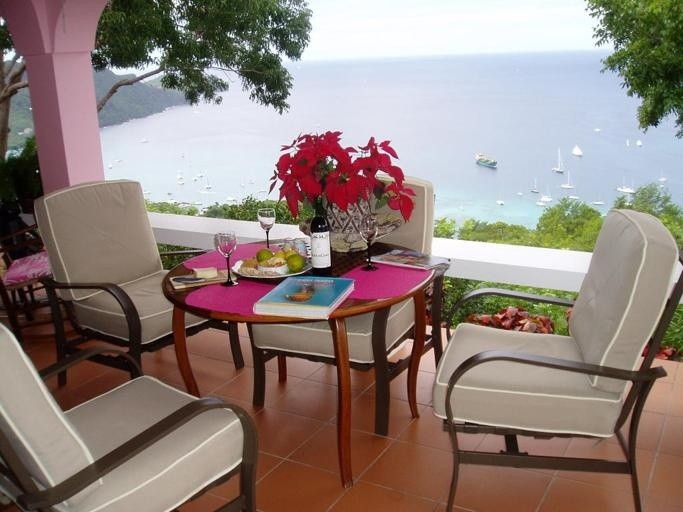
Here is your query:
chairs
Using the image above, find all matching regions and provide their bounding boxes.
[32,182,245,381]
[0,322,261,509]
[2,184,69,340]
[431,208,682,509]
[251,185,451,437]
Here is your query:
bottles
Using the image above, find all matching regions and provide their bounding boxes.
[282,237,307,262]
[311,194,331,278]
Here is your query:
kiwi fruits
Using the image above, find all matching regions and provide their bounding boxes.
[239,259,258,270]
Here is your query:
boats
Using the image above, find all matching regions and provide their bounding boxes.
[106,158,122,170]
[474,153,497,169]
[497,138,667,207]
[165,154,266,212]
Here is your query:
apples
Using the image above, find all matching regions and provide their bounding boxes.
[257,248,272,261]
[288,254,306,273]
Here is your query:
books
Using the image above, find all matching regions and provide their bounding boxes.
[366,249,451,270]
[253,276,354,320]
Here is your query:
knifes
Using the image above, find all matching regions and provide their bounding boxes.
[172,277,236,284]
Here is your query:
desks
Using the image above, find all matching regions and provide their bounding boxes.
[162,238,453,490]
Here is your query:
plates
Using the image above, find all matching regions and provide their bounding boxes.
[232,257,316,279]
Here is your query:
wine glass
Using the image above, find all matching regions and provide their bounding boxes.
[360,215,378,271]
[257,208,276,248]
[214,232,238,288]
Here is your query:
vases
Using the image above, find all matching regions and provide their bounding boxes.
[310,198,329,233]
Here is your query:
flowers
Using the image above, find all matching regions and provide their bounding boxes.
[271,132,418,223]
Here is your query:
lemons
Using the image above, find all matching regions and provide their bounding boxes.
[275,248,297,260]
